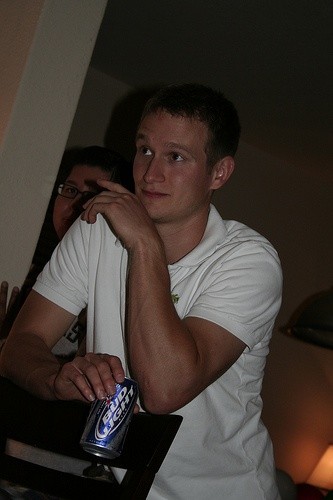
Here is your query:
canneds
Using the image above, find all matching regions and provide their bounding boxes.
[80,376,137,460]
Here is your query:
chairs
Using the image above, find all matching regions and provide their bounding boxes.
[0,373,183,500]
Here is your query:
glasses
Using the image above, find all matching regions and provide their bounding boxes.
[57,183,98,203]
